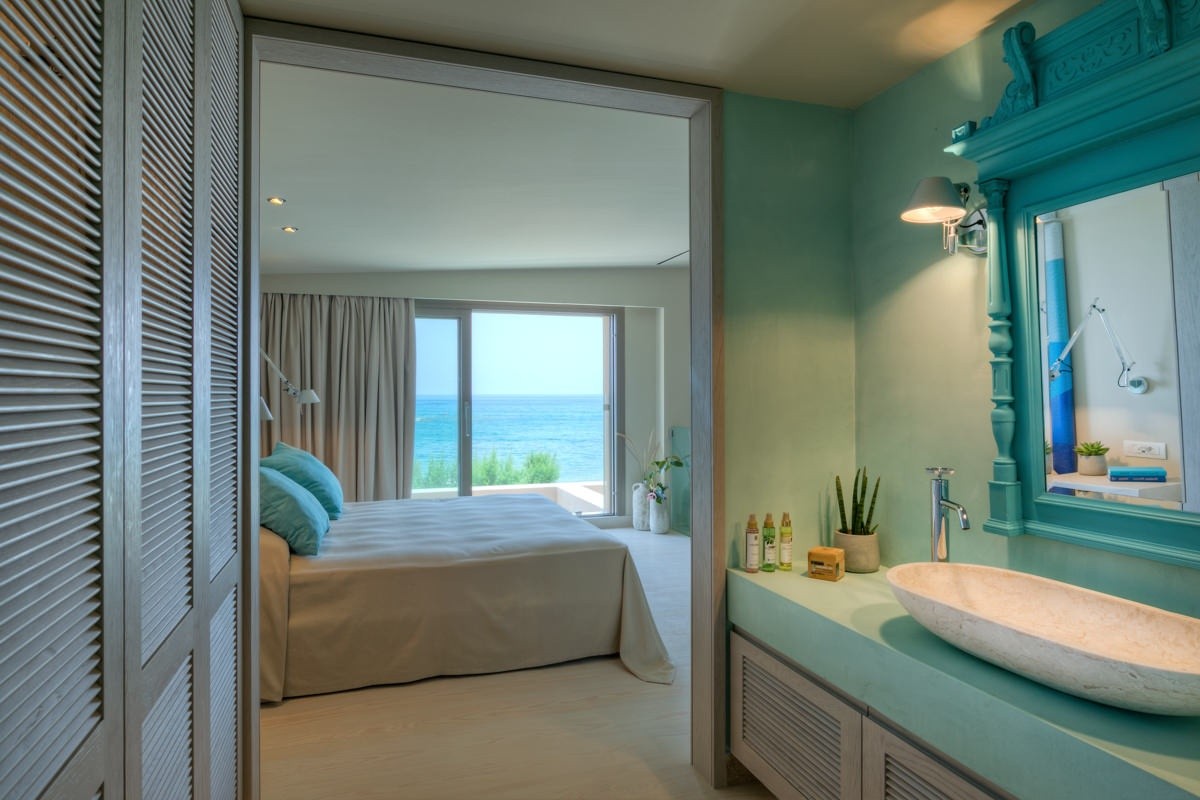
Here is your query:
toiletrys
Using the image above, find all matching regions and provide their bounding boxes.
[761,513,776,572]
[744,512,759,573]
[779,512,794,571]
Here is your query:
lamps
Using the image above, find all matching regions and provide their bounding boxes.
[899,175,987,253]
[260,348,320,406]
[1051,296,1147,394]
[260,397,274,423]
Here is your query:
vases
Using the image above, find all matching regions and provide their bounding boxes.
[649,499,670,533]
[629,481,651,531]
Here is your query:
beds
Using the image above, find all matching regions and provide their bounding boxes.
[260,495,678,704]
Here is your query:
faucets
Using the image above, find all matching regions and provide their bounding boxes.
[926,465,970,564]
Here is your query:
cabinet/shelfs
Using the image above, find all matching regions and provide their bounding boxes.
[728,625,998,800]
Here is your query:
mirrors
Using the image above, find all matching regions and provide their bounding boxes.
[1020,156,1200,530]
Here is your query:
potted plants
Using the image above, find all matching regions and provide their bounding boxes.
[1044,442,1055,475]
[1070,441,1110,476]
[832,465,883,574]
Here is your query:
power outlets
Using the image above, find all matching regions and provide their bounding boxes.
[1123,439,1169,461]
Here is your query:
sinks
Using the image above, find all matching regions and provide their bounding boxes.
[885,559,1200,716]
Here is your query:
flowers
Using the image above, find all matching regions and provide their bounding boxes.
[646,454,690,504]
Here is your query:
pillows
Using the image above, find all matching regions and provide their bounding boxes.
[261,440,343,522]
[259,468,332,557]
